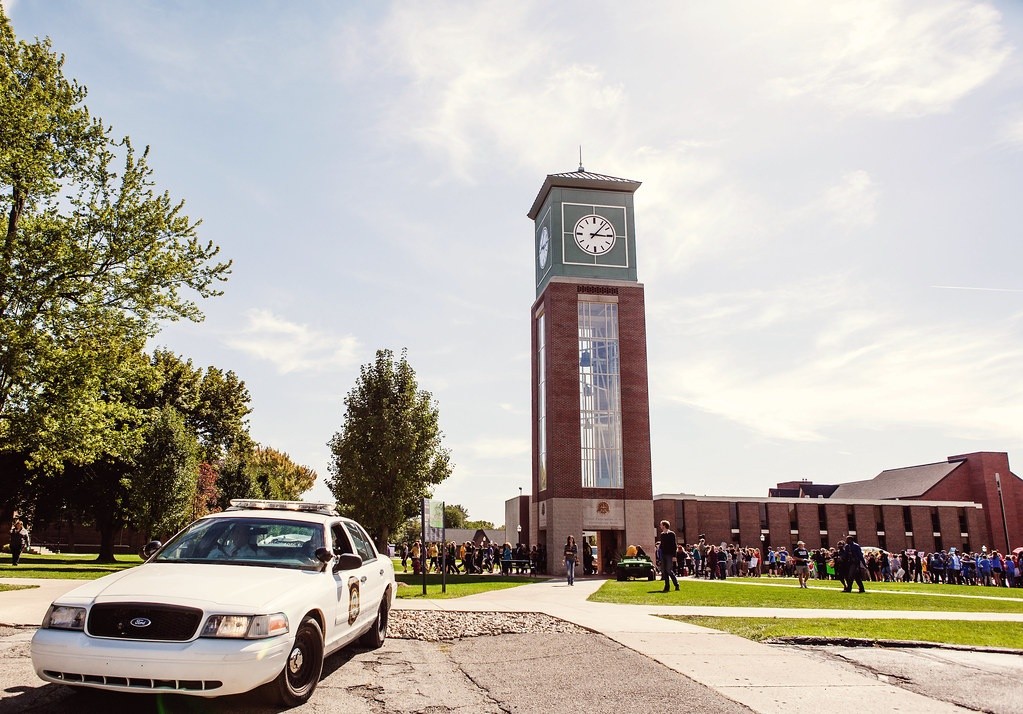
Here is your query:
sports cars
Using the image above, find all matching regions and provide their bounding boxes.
[616,555,656,581]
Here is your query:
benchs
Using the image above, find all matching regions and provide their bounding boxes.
[499,560,537,577]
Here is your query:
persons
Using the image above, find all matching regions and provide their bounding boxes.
[293,525,321,559]
[515,543,533,574]
[501,542,513,576]
[792,541,810,588]
[768,546,839,580]
[837,535,867,594]
[400,540,429,573]
[656,538,762,580]
[457,541,485,574]
[434,541,460,574]
[531,543,546,575]
[626,545,640,580]
[659,520,679,592]
[582,536,594,575]
[427,542,439,573]
[11,521,30,566]
[564,535,578,586]
[860,549,1023,588]
[485,542,502,574]
[207,523,255,559]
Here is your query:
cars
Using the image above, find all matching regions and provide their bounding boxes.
[590,545,598,574]
[30,498,398,710]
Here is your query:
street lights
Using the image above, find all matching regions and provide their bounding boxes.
[758,534,766,566]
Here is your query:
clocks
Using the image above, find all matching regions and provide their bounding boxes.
[575,216,614,254]
[538,229,549,267]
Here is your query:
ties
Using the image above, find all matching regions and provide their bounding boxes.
[231,548,239,557]
